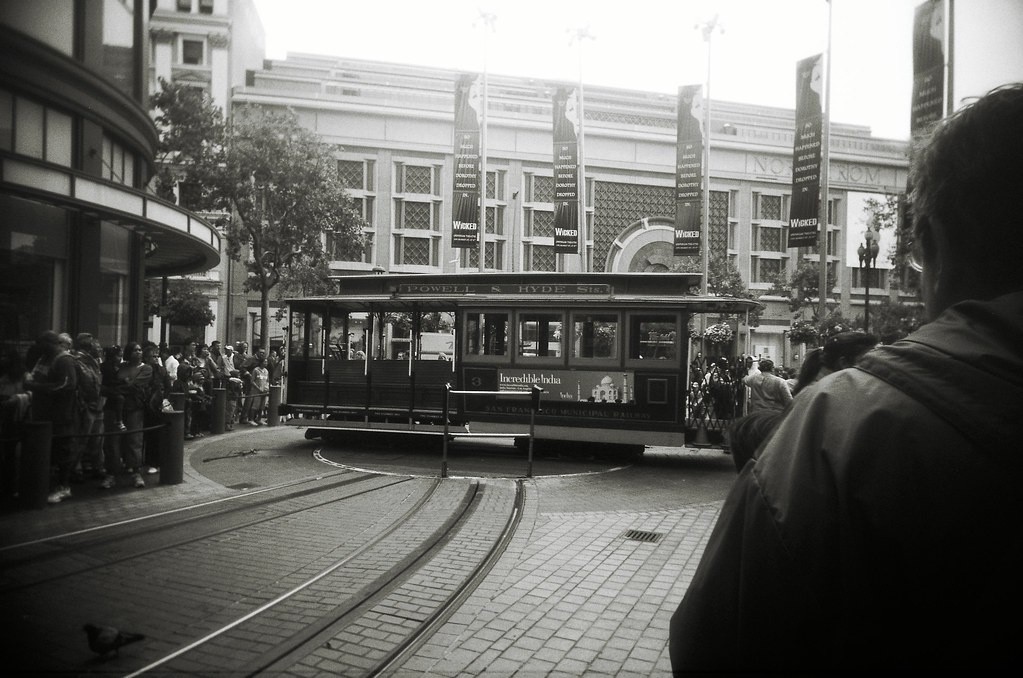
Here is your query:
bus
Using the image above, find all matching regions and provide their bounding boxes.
[277,263,764,460]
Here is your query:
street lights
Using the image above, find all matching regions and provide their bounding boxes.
[857,227,880,333]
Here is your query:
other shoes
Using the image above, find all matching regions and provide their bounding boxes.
[184,433,194,439]
[112,418,128,432]
[195,432,205,438]
[82,462,93,471]
[225,424,235,431]
[247,421,259,427]
[95,465,108,474]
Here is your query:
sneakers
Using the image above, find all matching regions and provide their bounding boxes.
[129,472,146,488]
[100,474,117,489]
[47,484,74,504]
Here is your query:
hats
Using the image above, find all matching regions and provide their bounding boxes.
[225,344,234,352]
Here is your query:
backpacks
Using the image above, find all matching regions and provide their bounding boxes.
[50,349,104,408]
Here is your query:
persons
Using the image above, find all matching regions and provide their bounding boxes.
[247,358,268,426]
[0,331,408,503]
[741,359,794,415]
[728,409,783,476]
[668,81,1023,678]
[173,362,197,439]
[687,350,797,418]
[788,331,879,397]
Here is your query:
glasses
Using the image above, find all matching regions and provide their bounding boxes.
[907,218,934,273]
[152,355,161,359]
